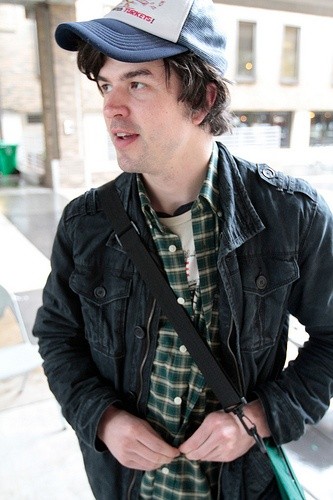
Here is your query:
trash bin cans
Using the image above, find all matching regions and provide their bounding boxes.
[0,143,21,177]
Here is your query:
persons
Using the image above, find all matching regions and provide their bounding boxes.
[29,0,333,500]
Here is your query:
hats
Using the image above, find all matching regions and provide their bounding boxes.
[55,0,227,77]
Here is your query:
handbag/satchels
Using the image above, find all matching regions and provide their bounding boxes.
[264,442,306,500]
